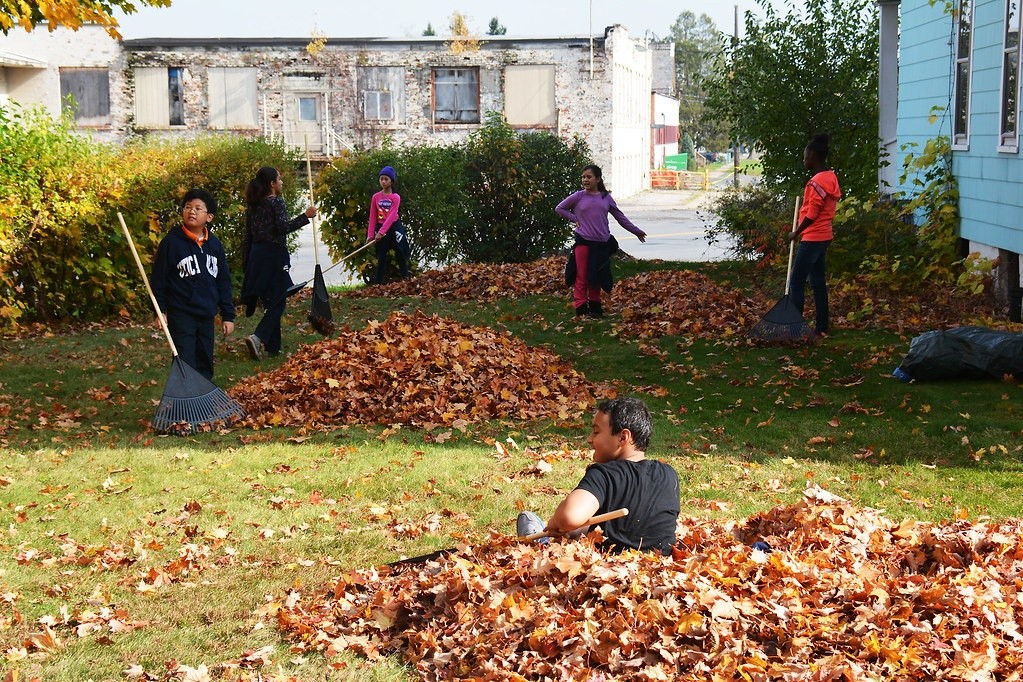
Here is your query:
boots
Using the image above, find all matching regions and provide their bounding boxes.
[576,302,589,319]
[588,300,602,316]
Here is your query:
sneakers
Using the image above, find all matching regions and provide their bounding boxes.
[245,334,262,361]
[517,511,549,544]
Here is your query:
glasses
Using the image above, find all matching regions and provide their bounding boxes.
[183,208,209,214]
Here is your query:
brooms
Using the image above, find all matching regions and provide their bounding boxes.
[382,508,628,578]
[285,135,380,341]
[116,210,248,436]
[746,197,813,343]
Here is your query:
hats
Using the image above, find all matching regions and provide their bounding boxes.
[379,166,396,181]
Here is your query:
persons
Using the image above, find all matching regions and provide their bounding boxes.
[243,167,317,363]
[150,190,237,382]
[789,134,841,334]
[515,398,678,556]
[365,165,410,285]
[556,165,647,317]
